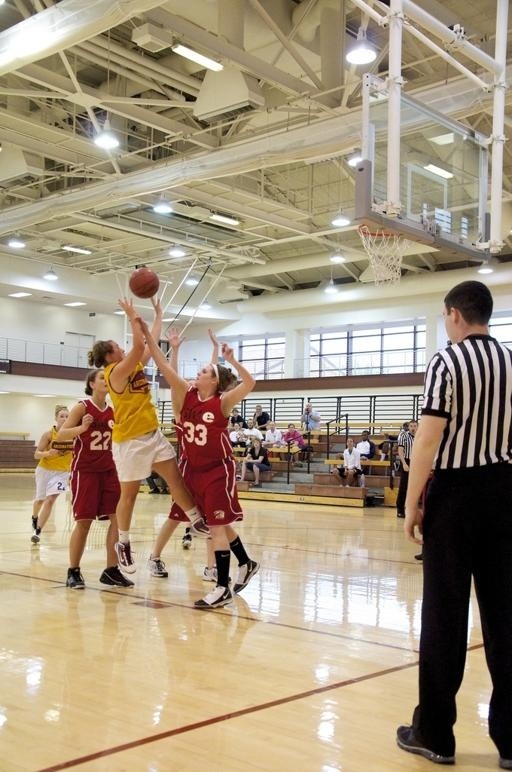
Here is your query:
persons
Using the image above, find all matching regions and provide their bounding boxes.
[31,405,75,544]
[280,424,306,467]
[240,418,265,453]
[252,404,271,430]
[56,369,137,591]
[356,429,375,459]
[331,437,362,487]
[136,315,261,611]
[261,422,283,446]
[379,421,409,462]
[228,423,243,446]
[396,420,418,517]
[89,295,212,573]
[302,404,322,431]
[231,408,249,428]
[238,437,271,485]
[395,279,511,771]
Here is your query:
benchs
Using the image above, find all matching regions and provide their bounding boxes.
[303,435,362,443]
[232,431,327,478]
[154,478,249,491]
[313,473,392,487]
[294,483,368,499]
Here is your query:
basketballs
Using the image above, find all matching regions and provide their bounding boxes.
[129,267,160,298]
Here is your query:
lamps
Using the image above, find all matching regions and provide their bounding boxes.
[7,237,26,249]
[209,213,240,225]
[92,118,120,150]
[42,270,58,280]
[328,250,344,263]
[168,247,186,257]
[475,261,492,274]
[345,151,361,166]
[60,245,92,255]
[323,279,337,294]
[344,36,379,65]
[152,199,173,214]
[198,300,210,309]
[422,163,453,179]
[329,214,348,226]
[186,278,199,285]
[171,44,224,72]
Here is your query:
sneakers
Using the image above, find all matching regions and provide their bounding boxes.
[114,541,136,574]
[190,517,211,540]
[339,484,343,487]
[100,566,135,589]
[499,756,512,770]
[66,567,85,589]
[182,533,192,547]
[397,513,405,517]
[396,726,456,764]
[233,559,260,593]
[160,489,167,494]
[31,527,41,543]
[148,488,159,494]
[345,485,350,487]
[31,516,38,529]
[194,585,233,608]
[146,553,168,577]
[202,567,217,583]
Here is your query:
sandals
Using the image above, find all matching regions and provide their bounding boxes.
[249,482,259,487]
